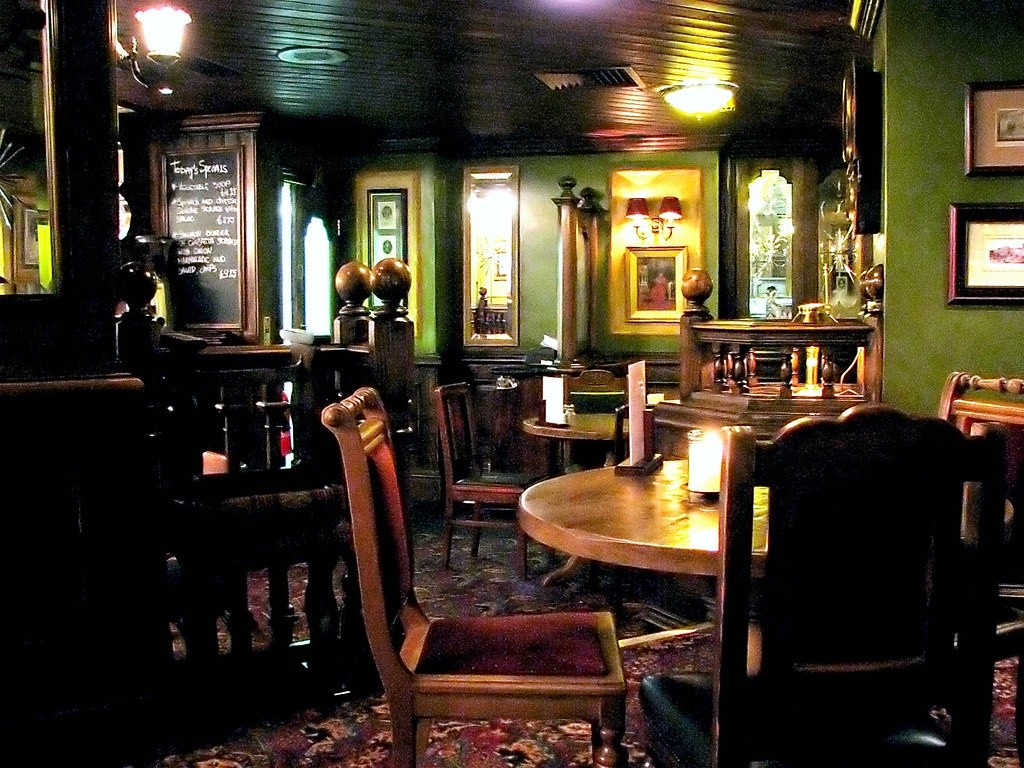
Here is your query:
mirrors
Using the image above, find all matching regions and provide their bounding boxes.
[0,0,64,297]
[463,164,520,347]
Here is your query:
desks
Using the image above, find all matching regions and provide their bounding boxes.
[521,413,630,587]
[520,458,1014,649]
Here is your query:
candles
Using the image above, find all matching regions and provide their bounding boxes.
[688,432,722,493]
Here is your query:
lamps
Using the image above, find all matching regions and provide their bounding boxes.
[626,195,683,241]
[121,5,193,95]
[661,79,739,116]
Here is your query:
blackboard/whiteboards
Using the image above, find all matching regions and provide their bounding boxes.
[159,146,246,332]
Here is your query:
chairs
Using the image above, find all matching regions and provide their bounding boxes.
[435,382,557,579]
[321,386,629,767]
[637,371,1024,768]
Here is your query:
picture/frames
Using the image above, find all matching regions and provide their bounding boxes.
[367,188,409,312]
[625,246,690,324]
[945,202,1024,306]
[964,80,1024,177]
[12,192,40,284]
